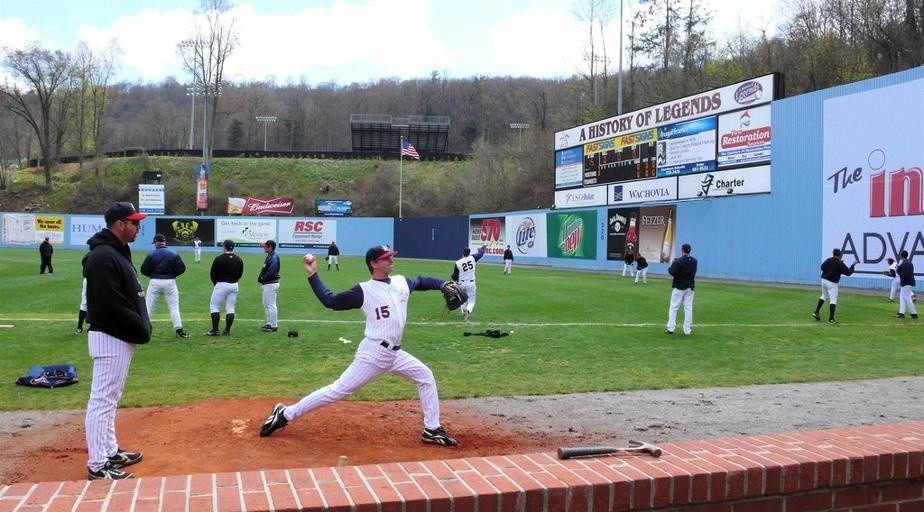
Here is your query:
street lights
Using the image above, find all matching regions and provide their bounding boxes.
[253,115,279,151]
[181,37,211,149]
[510,122,531,152]
[185,78,223,174]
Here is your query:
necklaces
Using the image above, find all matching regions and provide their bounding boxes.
[665,243,697,337]
[884,257,916,303]
[812,247,858,324]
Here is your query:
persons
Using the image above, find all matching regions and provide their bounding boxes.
[895,250,917,319]
[38,236,53,274]
[449,243,489,317]
[502,244,513,276]
[257,245,469,446]
[326,240,340,272]
[199,164,206,191]
[191,236,202,263]
[621,246,634,278]
[139,233,191,338]
[82,200,154,481]
[635,252,648,283]
[203,240,243,337]
[257,239,280,333]
[73,249,92,335]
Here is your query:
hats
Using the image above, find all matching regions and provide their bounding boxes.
[104,201,147,224]
[366,246,398,265]
[151,234,166,244]
[262,241,275,248]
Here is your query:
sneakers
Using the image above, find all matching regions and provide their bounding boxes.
[106,449,141,468]
[174,330,228,336]
[261,325,278,332]
[423,427,458,444]
[88,465,134,480]
[261,403,287,434]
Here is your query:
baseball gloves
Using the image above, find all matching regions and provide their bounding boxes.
[441,281,468,310]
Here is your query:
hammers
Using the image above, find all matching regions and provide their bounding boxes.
[558,439,662,460]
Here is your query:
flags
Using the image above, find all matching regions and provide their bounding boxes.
[400,139,419,161]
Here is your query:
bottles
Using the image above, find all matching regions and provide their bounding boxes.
[194,164,209,212]
[659,207,674,263]
[625,211,637,262]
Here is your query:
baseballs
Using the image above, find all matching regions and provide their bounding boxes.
[305,254,314,264]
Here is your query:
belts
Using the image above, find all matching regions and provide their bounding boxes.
[381,340,401,351]
